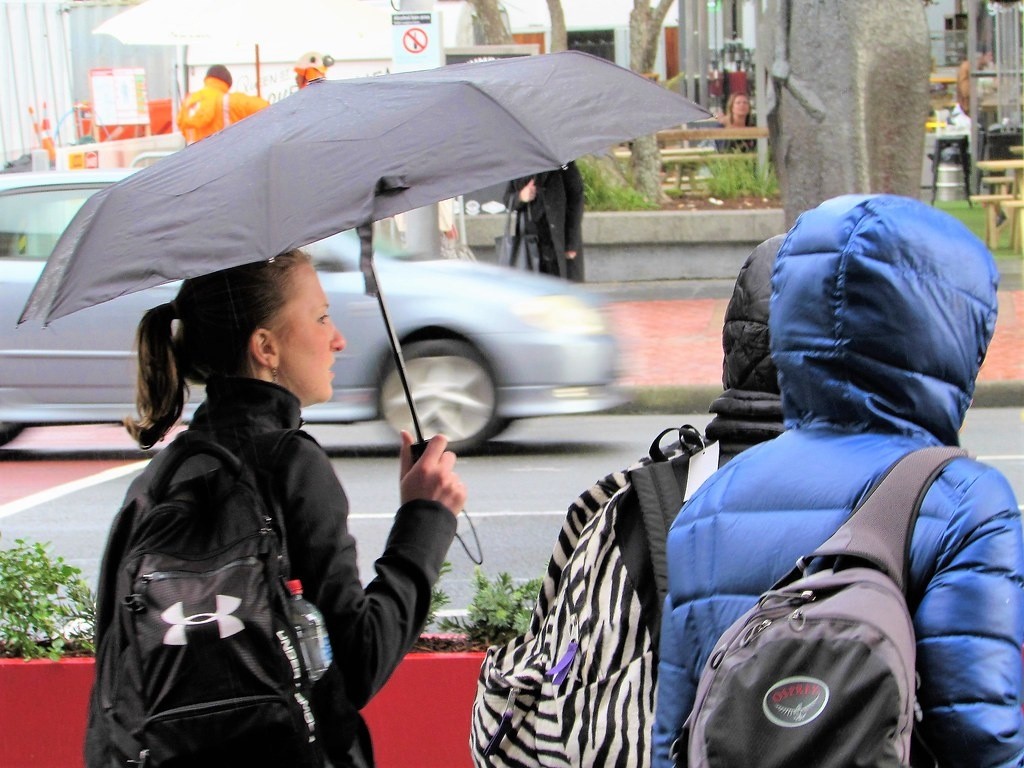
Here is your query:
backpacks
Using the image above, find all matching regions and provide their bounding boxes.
[469,422,704,768]
[83,428,375,768]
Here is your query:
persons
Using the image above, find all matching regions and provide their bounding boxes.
[607,232,784,768]
[697,91,759,154]
[955,41,999,133]
[652,194,1024,768]
[176,65,272,148]
[504,161,585,284]
[83,246,470,768]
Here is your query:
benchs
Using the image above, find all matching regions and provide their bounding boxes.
[967,177,1024,255]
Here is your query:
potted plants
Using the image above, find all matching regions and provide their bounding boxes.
[0,533,551,768]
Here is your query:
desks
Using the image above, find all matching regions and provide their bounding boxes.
[978,159,1024,197]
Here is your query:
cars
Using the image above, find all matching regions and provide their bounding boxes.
[0,170,625,455]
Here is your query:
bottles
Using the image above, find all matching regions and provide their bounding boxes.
[284,577,333,680]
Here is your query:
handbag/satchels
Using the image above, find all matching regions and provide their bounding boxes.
[669,446,969,768]
[495,193,540,274]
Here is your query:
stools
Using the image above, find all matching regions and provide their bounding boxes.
[930,134,972,207]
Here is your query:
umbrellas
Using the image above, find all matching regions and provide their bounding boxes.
[91,0,389,100]
[15,46,714,474]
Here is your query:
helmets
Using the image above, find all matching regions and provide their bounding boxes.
[294,51,334,80]
